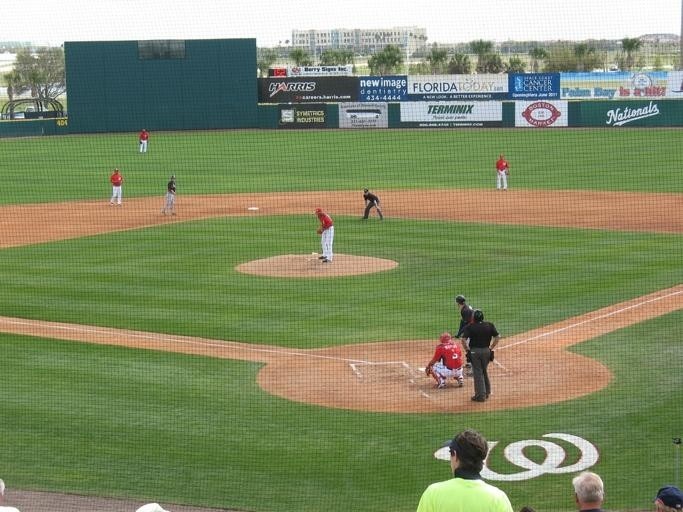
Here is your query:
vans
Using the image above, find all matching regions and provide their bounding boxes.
[24,106,48,113]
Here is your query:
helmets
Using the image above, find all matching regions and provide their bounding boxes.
[456,295,465,302]
[474,310,484,320]
[439,333,451,343]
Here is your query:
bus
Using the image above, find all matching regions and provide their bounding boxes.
[344,107,383,120]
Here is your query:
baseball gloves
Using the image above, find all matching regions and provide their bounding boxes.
[506,171,510,176]
[425,363,434,376]
[318,227,324,232]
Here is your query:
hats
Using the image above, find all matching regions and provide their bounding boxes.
[316,208,322,213]
[651,486,683,509]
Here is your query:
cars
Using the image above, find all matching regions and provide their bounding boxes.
[354,52,360,56]
[360,51,369,56]
[608,63,619,71]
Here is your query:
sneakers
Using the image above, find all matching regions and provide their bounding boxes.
[318,256,329,262]
[471,392,490,402]
[438,381,446,389]
[458,379,464,387]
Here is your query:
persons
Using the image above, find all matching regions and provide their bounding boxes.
[109,169,123,206]
[362,189,386,220]
[416,431,513,512]
[425,295,500,401]
[496,154,509,191]
[139,129,149,153]
[654,486,683,512]
[161,175,177,216]
[315,207,334,262]
[572,470,608,512]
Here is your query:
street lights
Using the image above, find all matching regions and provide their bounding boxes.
[278,40,284,65]
[284,39,299,65]
[407,31,434,76]
